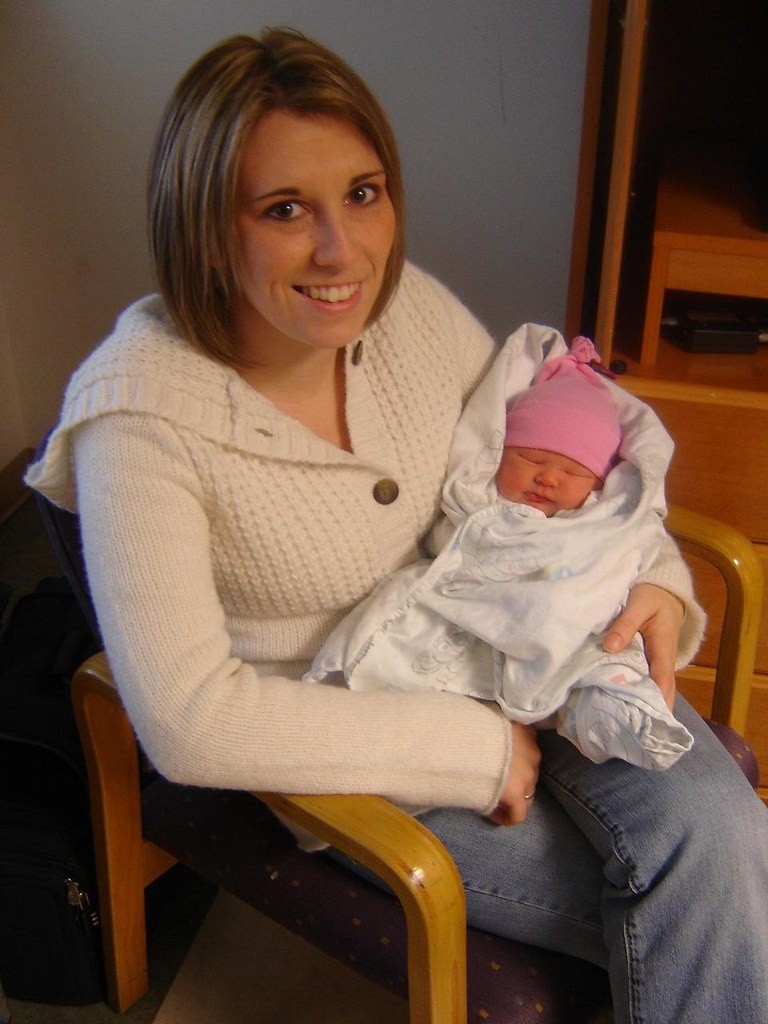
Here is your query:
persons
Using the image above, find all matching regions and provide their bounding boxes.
[20,31,768,1023]
[298,321,696,816]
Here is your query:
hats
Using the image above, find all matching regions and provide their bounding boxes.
[504,335,622,483]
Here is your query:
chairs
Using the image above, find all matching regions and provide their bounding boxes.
[30,416,762,1024]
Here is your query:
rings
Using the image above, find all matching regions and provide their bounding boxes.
[525,788,537,799]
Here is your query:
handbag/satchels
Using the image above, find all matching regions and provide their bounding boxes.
[1,585,160,1007]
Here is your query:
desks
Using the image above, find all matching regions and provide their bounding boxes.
[638,159,767,366]
